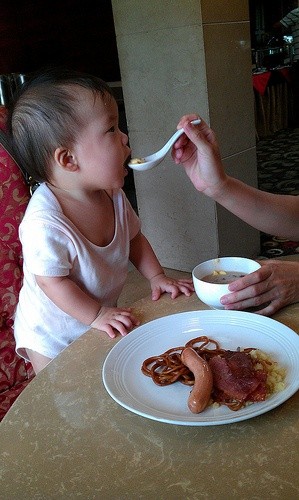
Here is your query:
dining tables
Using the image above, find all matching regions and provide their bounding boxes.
[0,254,299,500]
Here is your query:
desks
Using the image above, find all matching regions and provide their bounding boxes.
[251,67,289,140]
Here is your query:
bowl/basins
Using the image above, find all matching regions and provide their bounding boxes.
[192,257,261,312]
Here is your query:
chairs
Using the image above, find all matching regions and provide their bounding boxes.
[0,105,37,422]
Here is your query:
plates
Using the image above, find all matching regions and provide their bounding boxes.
[102,310,299,426]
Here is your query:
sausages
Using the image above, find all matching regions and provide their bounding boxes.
[181,347,212,413]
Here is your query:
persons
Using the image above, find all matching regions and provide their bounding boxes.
[12,69,195,376]
[271,7,299,126]
[170,113,299,315]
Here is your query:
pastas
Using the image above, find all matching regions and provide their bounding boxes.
[141,337,287,412]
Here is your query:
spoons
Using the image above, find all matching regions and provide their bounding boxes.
[127,119,202,172]
